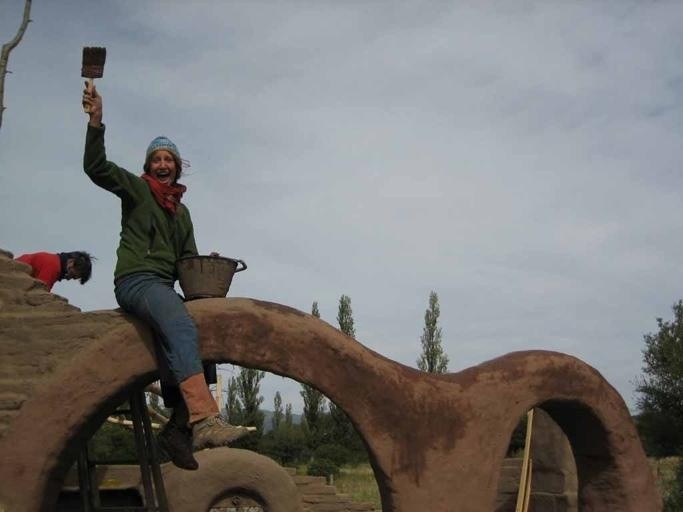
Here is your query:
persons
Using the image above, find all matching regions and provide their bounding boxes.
[80,80,251,472]
[13,250,92,294]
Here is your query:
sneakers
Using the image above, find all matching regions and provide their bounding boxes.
[191,415,249,448]
[157,424,198,470]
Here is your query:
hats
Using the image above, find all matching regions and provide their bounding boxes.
[145,135,179,164]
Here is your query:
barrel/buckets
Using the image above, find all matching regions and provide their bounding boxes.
[175,255,246,303]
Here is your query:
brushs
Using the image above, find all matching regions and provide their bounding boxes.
[81,47,106,114]
